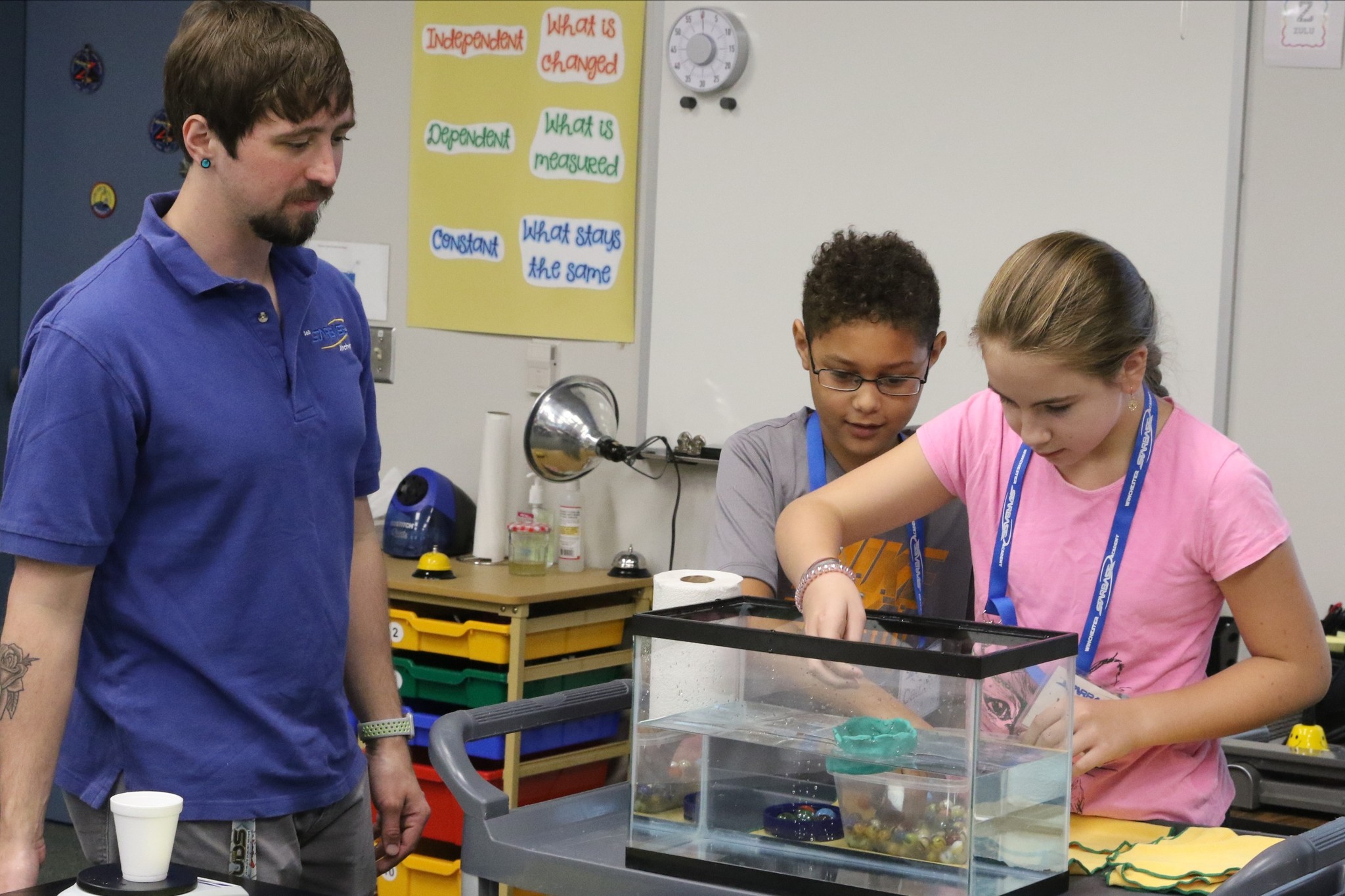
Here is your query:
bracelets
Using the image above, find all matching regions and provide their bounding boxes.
[796,557,856,615]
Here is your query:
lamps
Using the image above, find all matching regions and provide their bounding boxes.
[523,374,721,484]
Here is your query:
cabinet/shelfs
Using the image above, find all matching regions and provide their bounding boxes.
[386,548,659,896]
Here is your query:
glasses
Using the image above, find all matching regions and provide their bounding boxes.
[806,333,934,398]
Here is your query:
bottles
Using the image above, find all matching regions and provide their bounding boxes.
[556,481,585,573]
[507,522,551,576]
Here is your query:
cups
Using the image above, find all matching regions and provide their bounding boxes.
[109,791,183,881]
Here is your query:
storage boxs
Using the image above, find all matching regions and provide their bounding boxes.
[626,597,1080,895]
[371,599,638,896]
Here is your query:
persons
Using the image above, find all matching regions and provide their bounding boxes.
[774,233,1331,828]
[0,0,431,896]
[706,227,973,727]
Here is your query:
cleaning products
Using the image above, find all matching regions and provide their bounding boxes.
[516,471,585,572]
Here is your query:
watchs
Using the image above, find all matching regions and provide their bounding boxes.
[358,712,415,744]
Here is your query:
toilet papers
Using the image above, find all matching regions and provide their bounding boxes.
[649,569,745,721]
[472,410,514,564]
